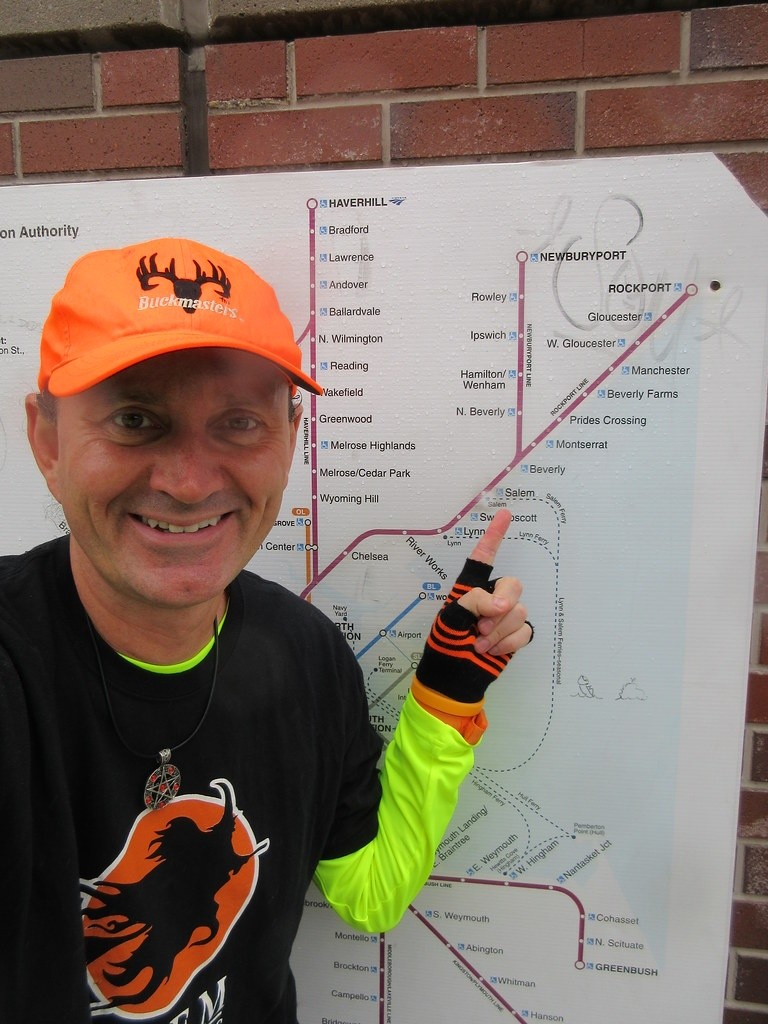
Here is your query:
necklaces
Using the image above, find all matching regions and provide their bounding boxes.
[81,609,219,811]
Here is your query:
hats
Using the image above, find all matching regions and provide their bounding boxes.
[37,238,324,398]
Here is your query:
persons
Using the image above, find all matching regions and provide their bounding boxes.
[0,238,536,1024]
